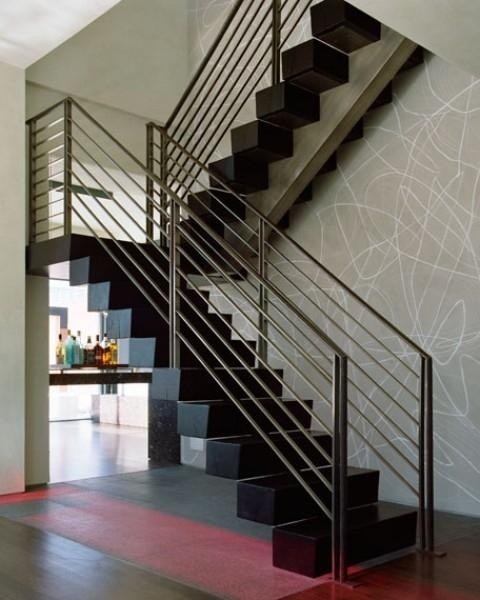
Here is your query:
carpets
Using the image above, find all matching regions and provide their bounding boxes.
[0,464,480,600]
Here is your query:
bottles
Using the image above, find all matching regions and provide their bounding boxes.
[56,329,119,369]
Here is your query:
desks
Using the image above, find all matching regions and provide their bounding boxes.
[48,364,151,384]
